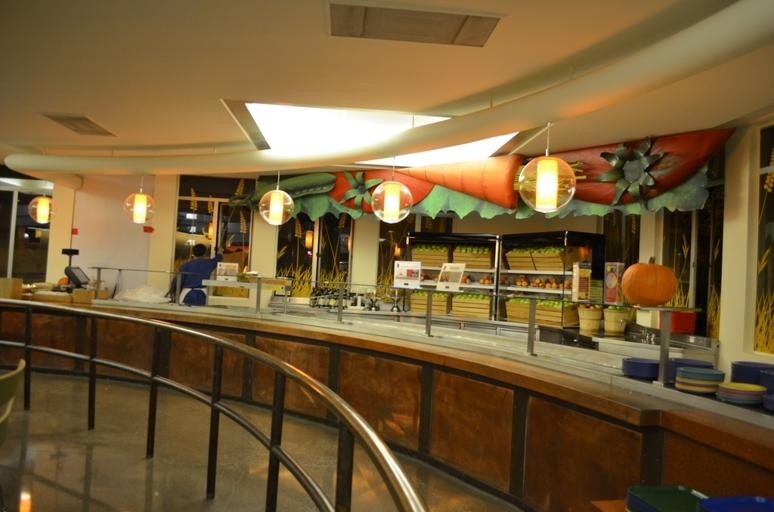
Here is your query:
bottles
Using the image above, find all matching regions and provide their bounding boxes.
[311,287,347,308]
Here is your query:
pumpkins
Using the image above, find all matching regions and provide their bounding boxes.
[622,256,677,306]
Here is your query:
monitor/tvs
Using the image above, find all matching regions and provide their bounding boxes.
[64,266,90,288]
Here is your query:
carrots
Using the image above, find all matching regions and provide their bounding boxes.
[395,154,523,209]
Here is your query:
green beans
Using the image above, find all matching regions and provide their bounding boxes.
[250,173,336,203]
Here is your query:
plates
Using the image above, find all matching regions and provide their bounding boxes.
[622,358,773,411]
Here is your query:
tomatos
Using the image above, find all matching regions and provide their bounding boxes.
[533,128,737,206]
[329,169,434,213]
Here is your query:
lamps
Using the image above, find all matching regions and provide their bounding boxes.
[518,120,576,212]
[371,166,413,225]
[259,171,294,226]
[28,193,52,224]
[122,176,155,224]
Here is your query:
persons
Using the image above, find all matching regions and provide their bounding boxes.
[168,244,225,307]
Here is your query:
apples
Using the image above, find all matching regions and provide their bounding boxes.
[414,245,623,311]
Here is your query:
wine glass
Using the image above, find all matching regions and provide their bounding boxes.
[368,298,379,310]
[390,297,401,312]
